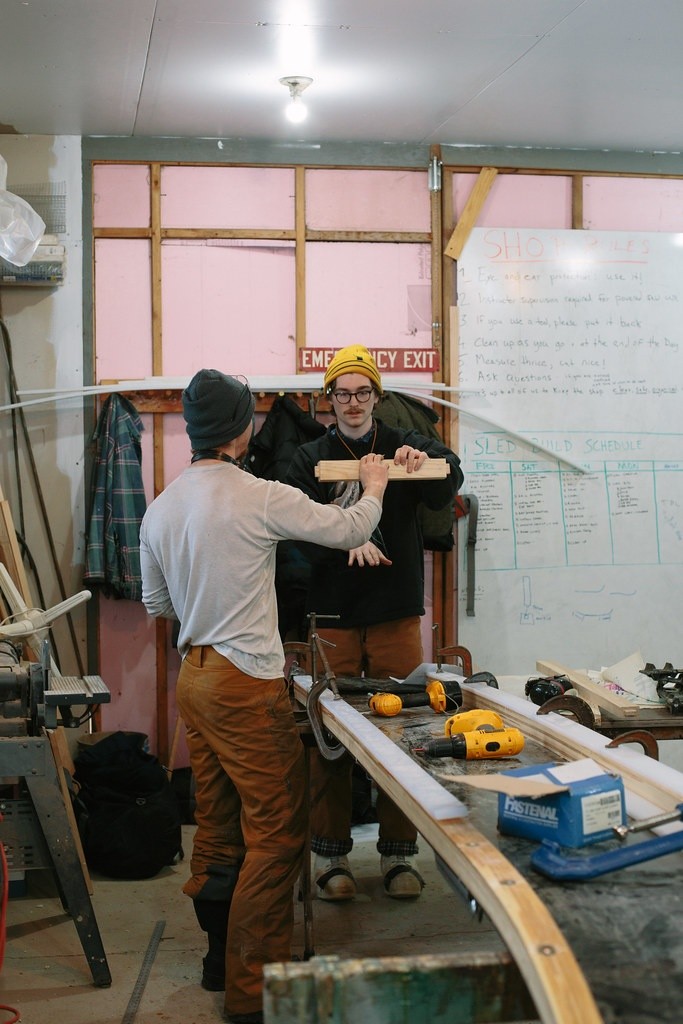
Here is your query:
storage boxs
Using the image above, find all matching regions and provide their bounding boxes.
[434,761,625,849]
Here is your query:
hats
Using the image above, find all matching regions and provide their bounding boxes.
[324,343,383,395]
[182,368,255,451]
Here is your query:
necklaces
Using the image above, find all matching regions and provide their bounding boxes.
[334,416,377,459]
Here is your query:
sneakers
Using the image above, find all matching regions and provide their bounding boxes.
[201,949,225,991]
[228,1010,262,1024]
[313,853,357,902]
[380,854,423,898]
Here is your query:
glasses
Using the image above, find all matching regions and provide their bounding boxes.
[332,387,375,404]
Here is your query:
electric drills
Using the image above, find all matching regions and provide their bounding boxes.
[410,709,524,760]
[368,681,462,716]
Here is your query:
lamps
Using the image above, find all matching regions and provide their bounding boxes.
[278,76,313,123]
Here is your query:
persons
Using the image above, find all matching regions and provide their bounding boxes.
[282,342,464,899]
[138,367,391,1023]
